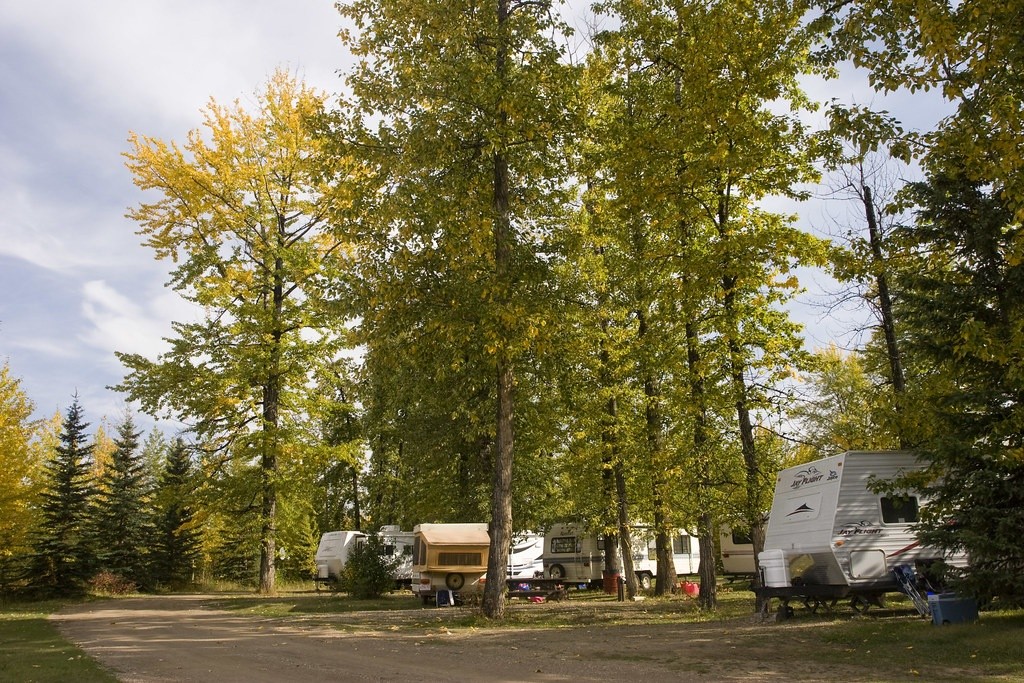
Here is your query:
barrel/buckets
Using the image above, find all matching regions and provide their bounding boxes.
[602,569,620,593]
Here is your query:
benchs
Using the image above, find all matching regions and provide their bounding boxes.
[505,591,550,603]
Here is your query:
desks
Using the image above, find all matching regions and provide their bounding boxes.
[505,577,568,602]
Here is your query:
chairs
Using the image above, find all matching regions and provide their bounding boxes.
[892,557,948,617]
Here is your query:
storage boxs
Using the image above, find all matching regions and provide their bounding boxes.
[928,592,979,625]
